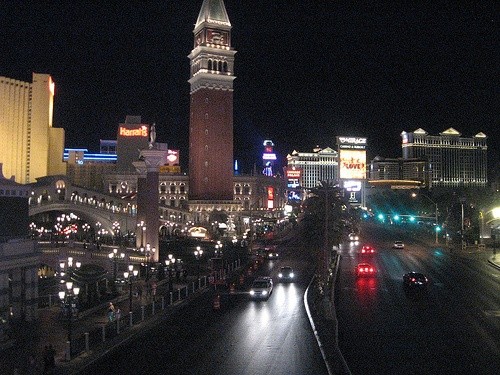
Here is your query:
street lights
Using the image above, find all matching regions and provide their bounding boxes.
[59,257,82,289]
[123,265,139,317]
[139,243,157,283]
[411,193,439,245]
[192,246,205,280]
[58,280,80,356]
[108,249,125,283]
[164,254,176,295]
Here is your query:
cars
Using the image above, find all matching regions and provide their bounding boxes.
[393,241,405,249]
[403,271,429,287]
[277,266,295,282]
[248,277,273,299]
[360,209,441,233]
[250,248,280,261]
[356,262,377,278]
[361,245,375,256]
[347,232,361,242]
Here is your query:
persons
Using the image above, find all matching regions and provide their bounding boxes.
[138,281,145,296]
[106,302,120,322]
[41,343,57,369]
